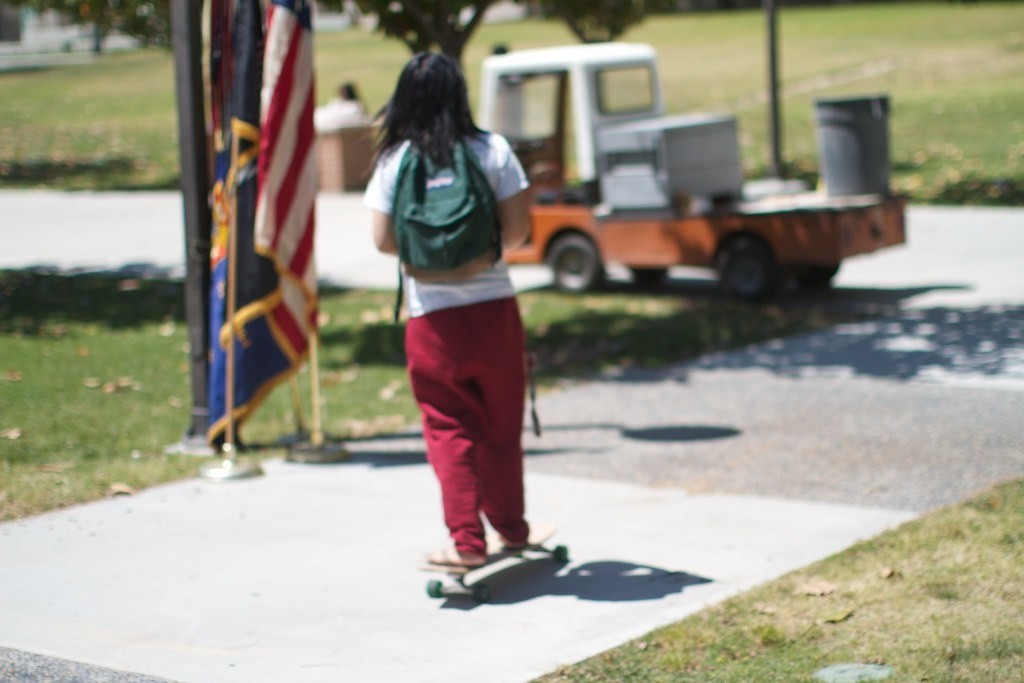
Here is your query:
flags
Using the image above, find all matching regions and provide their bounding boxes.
[201,0,319,445]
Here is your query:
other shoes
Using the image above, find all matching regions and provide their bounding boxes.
[419,552,486,572]
[502,540,529,552]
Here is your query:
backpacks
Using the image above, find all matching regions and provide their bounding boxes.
[390,135,501,270]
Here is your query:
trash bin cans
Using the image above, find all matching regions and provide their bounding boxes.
[814,95,891,196]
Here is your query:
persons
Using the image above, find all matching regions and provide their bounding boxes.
[366,51,530,566]
[312,82,372,136]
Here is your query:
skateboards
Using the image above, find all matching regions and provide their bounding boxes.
[428,535,569,605]
